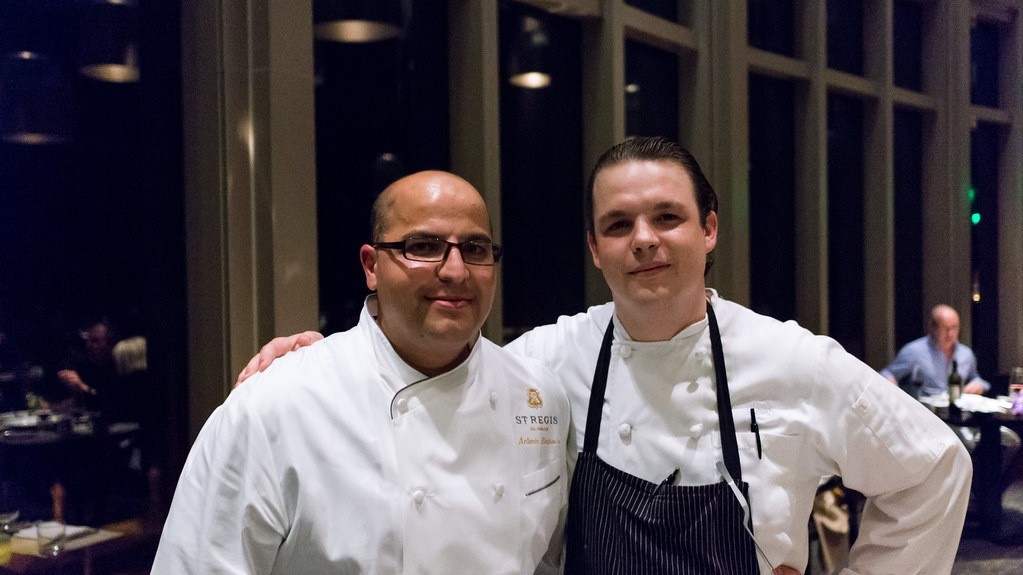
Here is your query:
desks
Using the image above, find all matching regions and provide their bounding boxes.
[0,524,126,575]
[929,398,1023,547]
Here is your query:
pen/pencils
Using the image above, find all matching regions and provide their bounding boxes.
[648,468,680,503]
[750,408,762,458]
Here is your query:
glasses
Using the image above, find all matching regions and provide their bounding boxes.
[373,236,504,266]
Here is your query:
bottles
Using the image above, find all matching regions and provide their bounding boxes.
[1009,367,1023,404]
[947,360,961,411]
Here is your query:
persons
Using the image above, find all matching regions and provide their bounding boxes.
[237,136,973,575]
[149,171,572,575]
[880,304,991,397]
[57,320,148,421]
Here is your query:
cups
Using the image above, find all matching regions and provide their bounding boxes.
[27,395,39,416]
[36,518,65,557]
